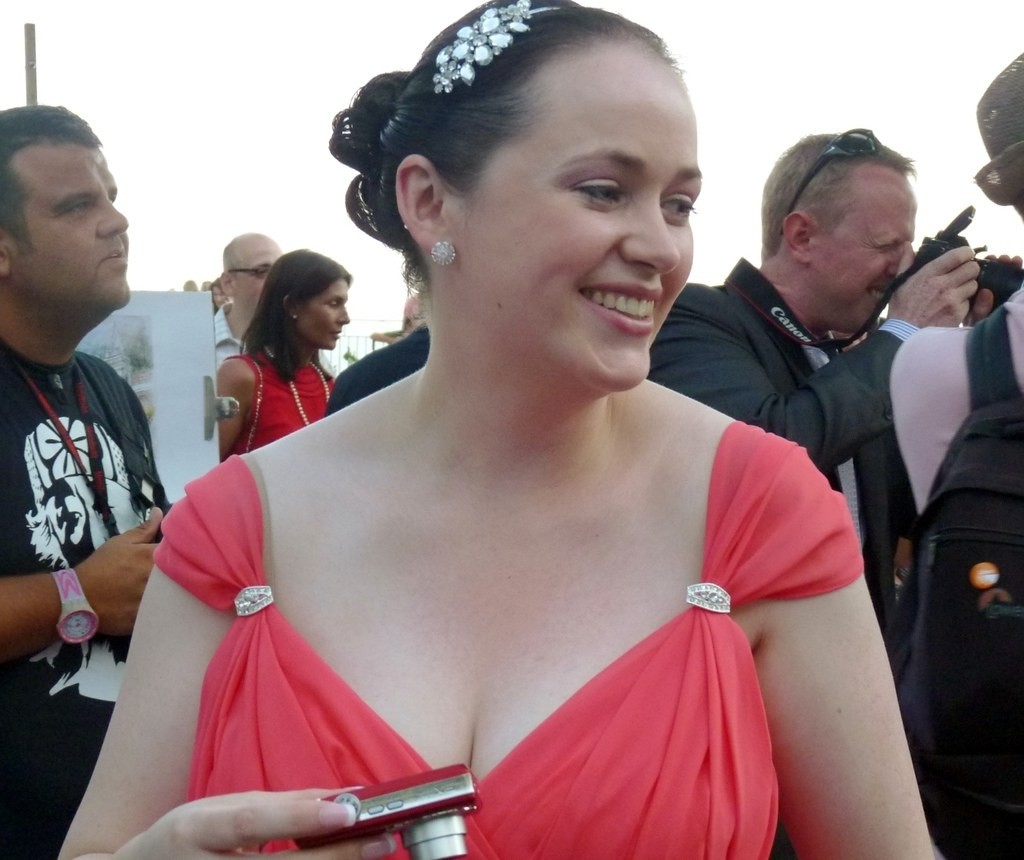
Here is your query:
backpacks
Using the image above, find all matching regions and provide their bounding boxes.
[886,305,1024,860]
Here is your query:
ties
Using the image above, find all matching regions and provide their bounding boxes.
[803,344,861,546]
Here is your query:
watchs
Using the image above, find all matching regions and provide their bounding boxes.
[52,569,100,644]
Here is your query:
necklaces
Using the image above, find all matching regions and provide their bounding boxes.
[264,344,331,426]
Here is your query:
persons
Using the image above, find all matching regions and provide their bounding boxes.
[59,0,942,860]
[891,52,1023,859]
[2,105,167,859]
[211,233,431,460]
[647,129,1020,648]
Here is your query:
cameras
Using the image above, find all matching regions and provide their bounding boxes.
[908,205,1024,317]
[291,763,482,860]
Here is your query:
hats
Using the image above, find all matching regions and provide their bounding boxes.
[975,52,1024,206]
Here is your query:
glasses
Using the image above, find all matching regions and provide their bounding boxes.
[229,264,271,279]
[779,128,883,236]
